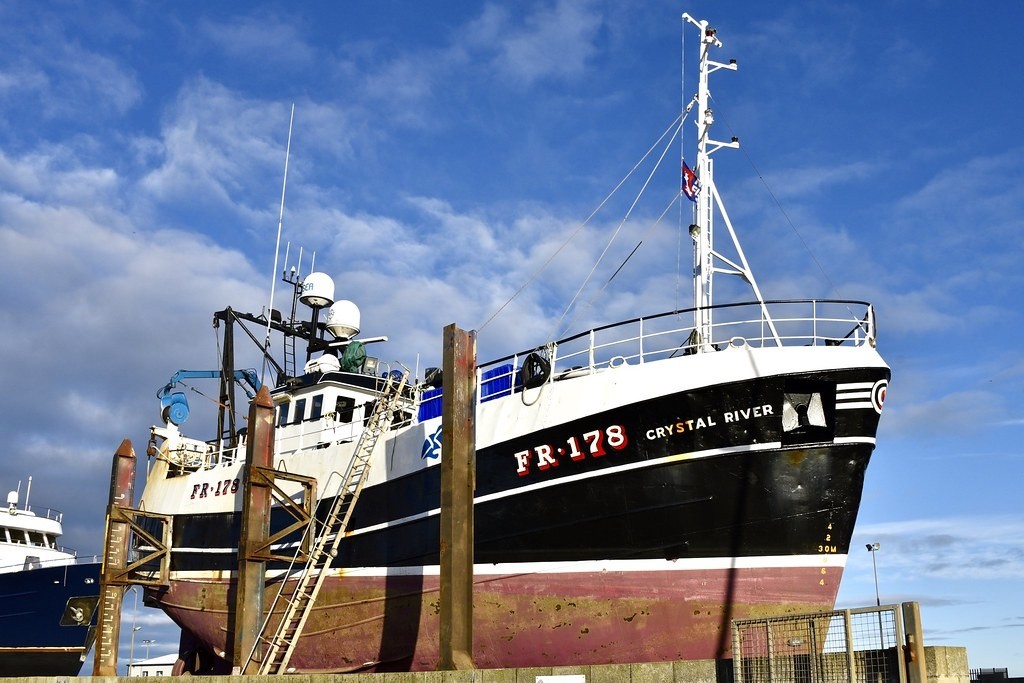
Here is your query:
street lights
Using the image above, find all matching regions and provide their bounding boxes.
[130,588,138,675]
[141,640,157,660]
[867,542,885,649]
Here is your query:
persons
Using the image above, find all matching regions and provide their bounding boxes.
[336,397,352,422]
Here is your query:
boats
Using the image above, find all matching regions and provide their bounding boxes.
[129,9,893,675]
[0,475,139,677]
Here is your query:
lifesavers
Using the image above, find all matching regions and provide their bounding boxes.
[522,353,551,389]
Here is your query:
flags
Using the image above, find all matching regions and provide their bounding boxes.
[682,157,703,202]
